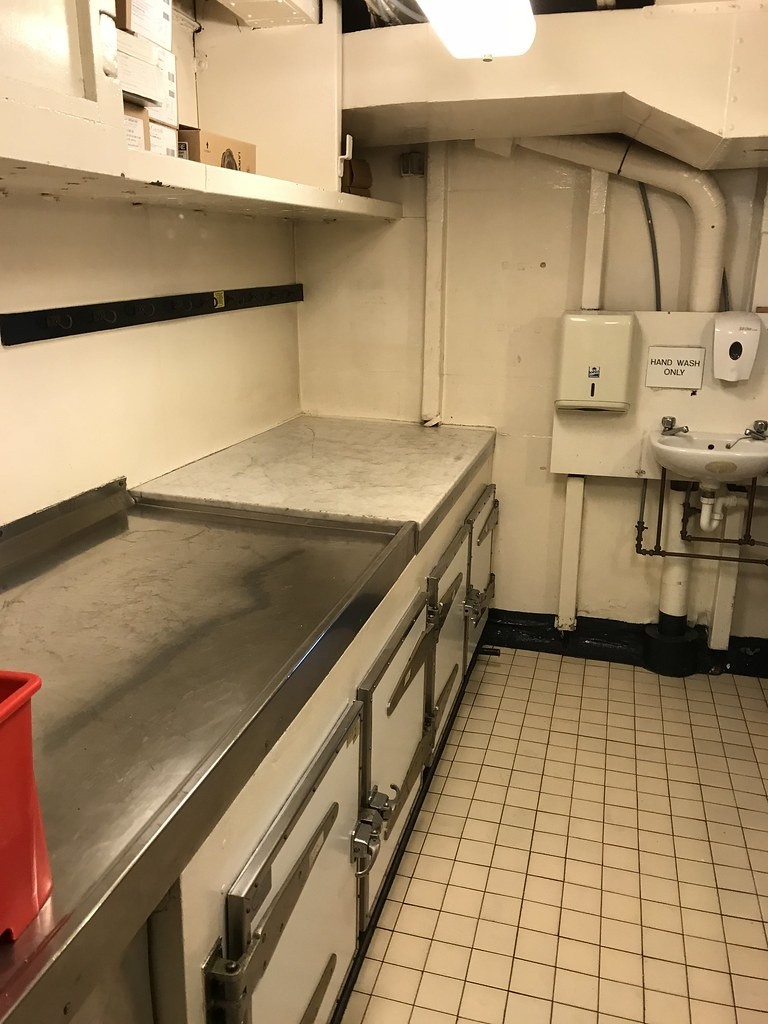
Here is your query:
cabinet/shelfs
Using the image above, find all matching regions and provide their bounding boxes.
[148,554,433,1024]
[416,438,499,784]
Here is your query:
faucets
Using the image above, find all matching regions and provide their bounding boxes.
[661,416,689,436]
[745,420,768,440]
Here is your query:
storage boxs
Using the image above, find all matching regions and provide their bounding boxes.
[116,28,164,108]
[178,123,257,174]
[115,0,173,51]
[127,31,179,129]
[149,121,178,157]
[123,100,151,151]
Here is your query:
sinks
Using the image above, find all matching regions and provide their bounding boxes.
[650,430,767,481]
